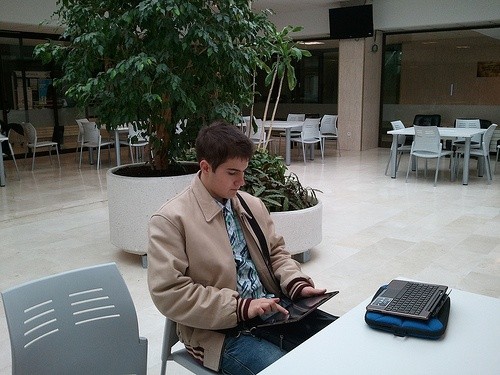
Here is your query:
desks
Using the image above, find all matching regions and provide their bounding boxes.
[256,278,500,375]
[387,126,489,185]
[0,133,10,187]
[99,122,129,165]
[239,121,305,166]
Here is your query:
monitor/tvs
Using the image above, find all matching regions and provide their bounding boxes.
[329,3,373,39]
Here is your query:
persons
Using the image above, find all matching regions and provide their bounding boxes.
[147,122,339,375]
[52,95,64,153]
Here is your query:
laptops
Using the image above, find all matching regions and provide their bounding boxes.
[366,279,450,320]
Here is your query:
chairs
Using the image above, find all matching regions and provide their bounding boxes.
[0,120,62,181]
[232,113,338,166]
[384,114,500,186]
[1,262,149,375]
[73,116,187,172]
[159,316,220,375]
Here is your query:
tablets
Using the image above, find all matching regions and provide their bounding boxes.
[256,291,339,328]
